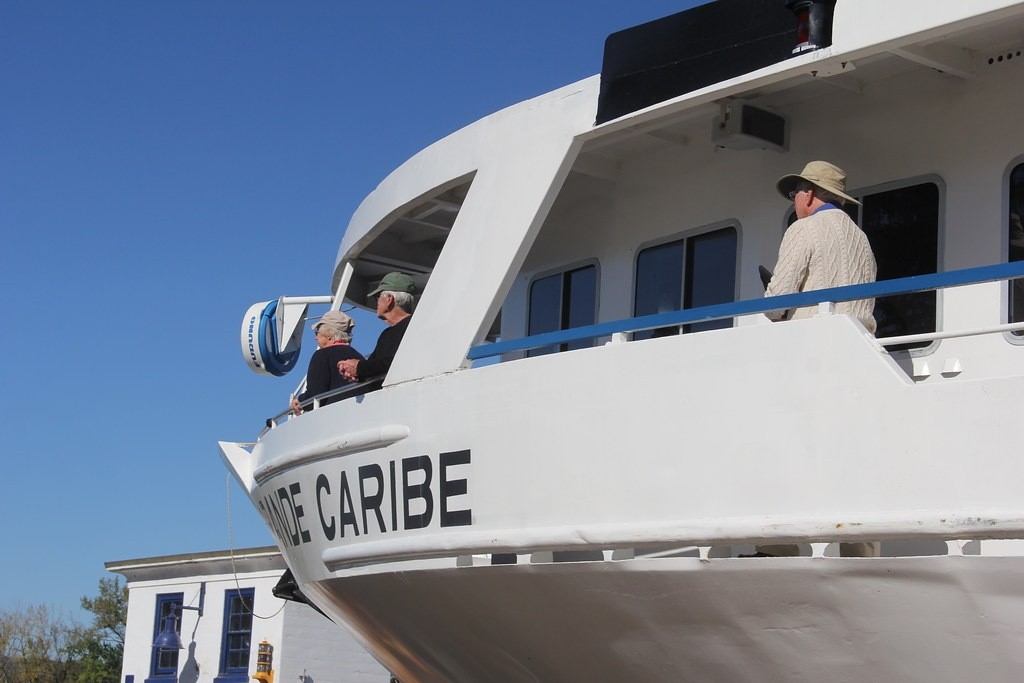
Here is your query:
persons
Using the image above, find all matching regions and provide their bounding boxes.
[737,160,876,558]
[290,310,366,416]
[337,272,416,395]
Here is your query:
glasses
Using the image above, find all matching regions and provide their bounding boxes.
[789,190,807,199]
[375,295,386,298]
[315,331,320,335]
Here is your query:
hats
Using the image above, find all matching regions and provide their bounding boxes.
[367,271,416,298]
[776,160,862,205]
[311,310,355,332]
